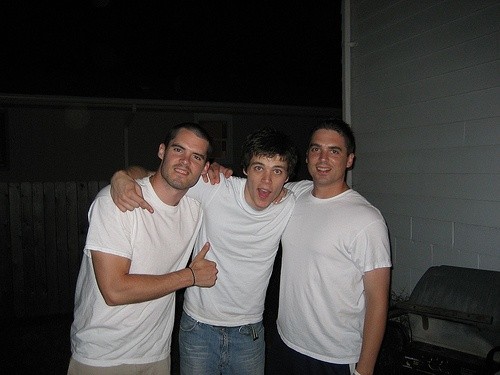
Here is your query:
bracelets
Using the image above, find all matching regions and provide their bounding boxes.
[353,369,360,375]
[186,266,195,285]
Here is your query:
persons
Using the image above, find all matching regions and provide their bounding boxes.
[201,118,393,375]
[67,126,286,375]
[110,129,296,375]
